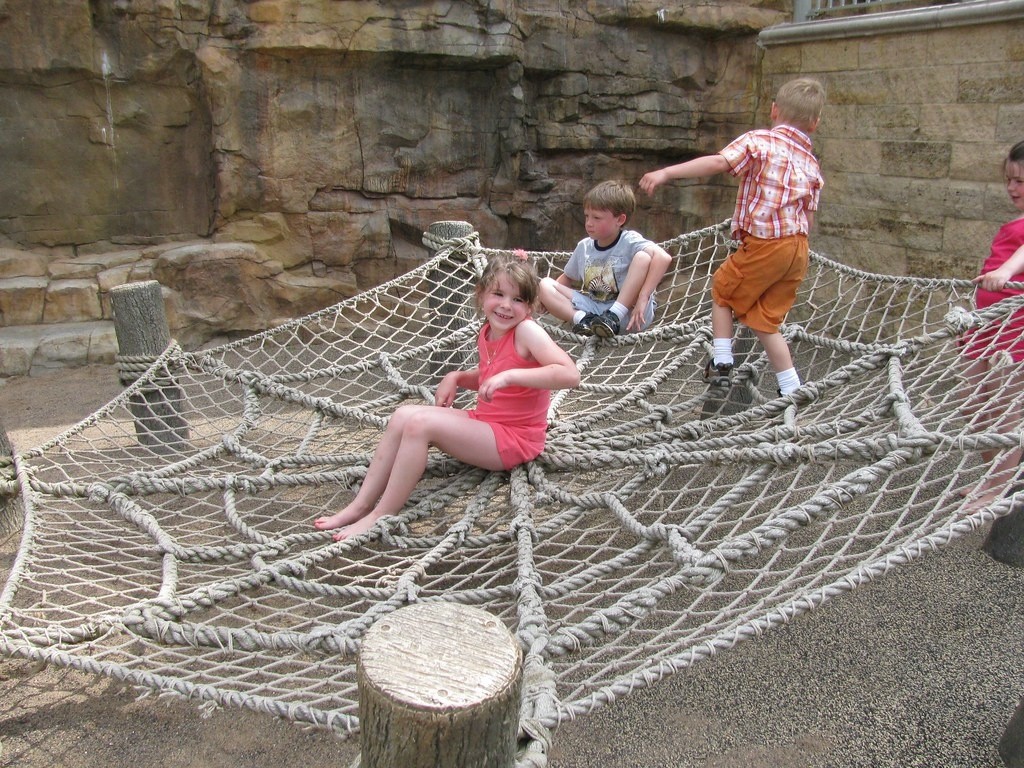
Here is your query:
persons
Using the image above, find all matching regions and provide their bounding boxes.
[637,77,827,396]
[539,178,674,337]
[312,250,584,542]
[958,135,1024,511]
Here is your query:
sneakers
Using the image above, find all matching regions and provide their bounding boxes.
[572,312,597,336]
[592,309,618,338]
[702,359,733,387]
